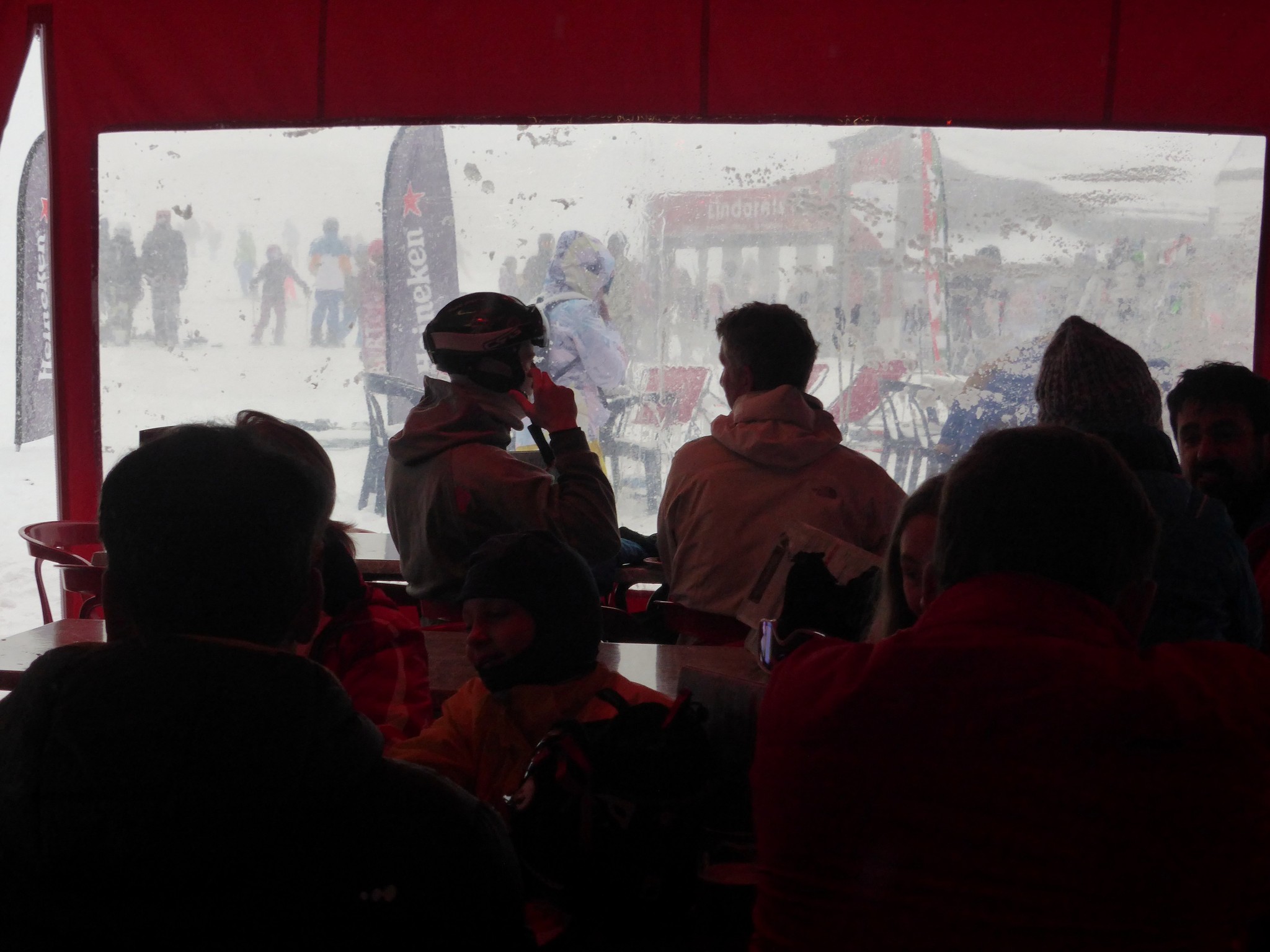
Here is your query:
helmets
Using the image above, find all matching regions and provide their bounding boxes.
[423,296,547,393]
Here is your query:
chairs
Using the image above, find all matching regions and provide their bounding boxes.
[24,521,107,625]
[354,369,959,516]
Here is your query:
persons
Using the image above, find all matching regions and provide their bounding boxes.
[749,425,1270,952]
[674,246,1005,376]
[1067,239,1147,353]
[1167,361,1270,656]
[515,231,630,478]
[1035,317,1262,654]
[384,291,623,629]
[883,474,947,637]
[236,411,432,741]
[656,300,910,628]
[237,217,387,375]
[99,209,188,352]
[388,513,674,817]
[602,231,632,343]
[0,424,543,952]
[498,232,555,305]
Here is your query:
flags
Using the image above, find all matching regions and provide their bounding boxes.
[921,127,952,379]
[14,132,55,452]
[383,125,460,425]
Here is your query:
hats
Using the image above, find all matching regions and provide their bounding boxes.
[456,529,601,684]
[1037,316,1164,436]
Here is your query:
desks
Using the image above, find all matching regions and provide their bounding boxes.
[0,617,771,698]
[356,560,668,586]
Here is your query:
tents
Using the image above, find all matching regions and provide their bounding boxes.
[648,162,839,265]
